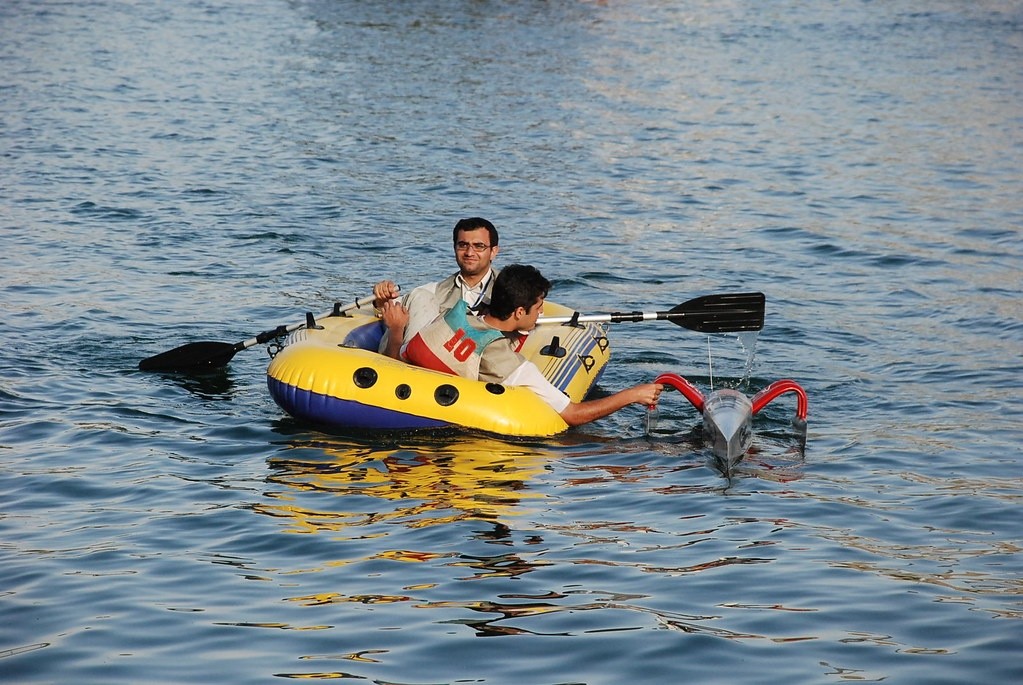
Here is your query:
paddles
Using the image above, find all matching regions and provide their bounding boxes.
[139,282,401,370]
[536,292,767,333]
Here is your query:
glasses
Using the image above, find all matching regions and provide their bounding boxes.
[454,241,491,252]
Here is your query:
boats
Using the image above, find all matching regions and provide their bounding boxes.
[266,298,611,441]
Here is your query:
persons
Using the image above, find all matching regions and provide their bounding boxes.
[381,264,665,426]
[372,217,500,384]
